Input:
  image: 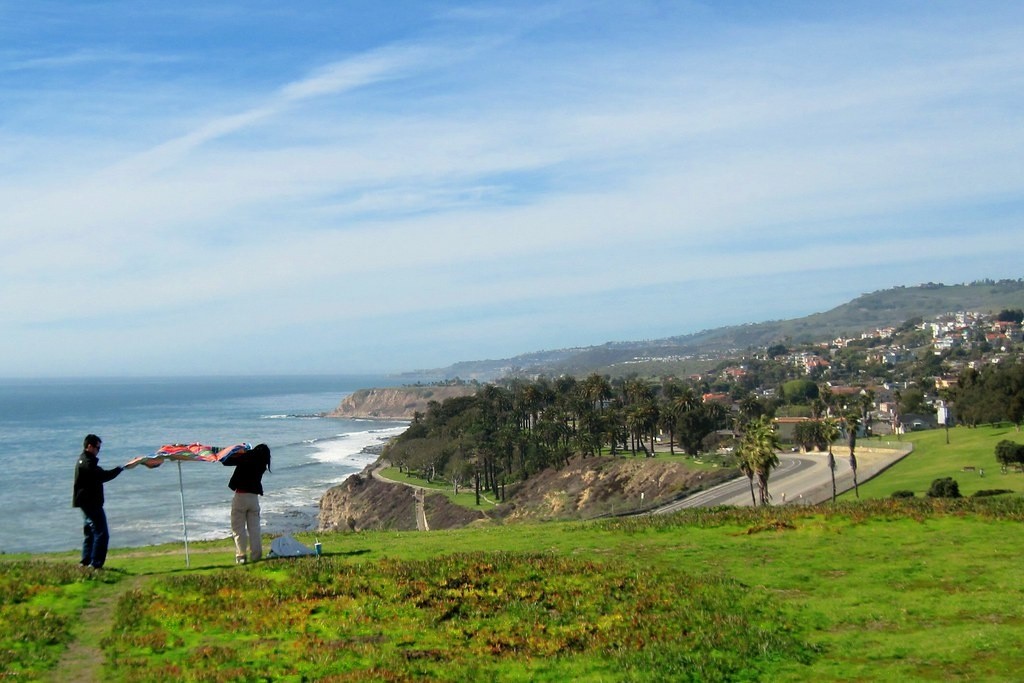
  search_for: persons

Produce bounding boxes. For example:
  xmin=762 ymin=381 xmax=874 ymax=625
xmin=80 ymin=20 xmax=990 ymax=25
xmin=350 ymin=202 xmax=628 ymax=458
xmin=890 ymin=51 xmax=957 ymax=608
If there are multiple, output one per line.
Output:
xmin=220 ymin=444 xmax=272 ymax=564
xmin=72 ymin=434 xmax=126 ymax=571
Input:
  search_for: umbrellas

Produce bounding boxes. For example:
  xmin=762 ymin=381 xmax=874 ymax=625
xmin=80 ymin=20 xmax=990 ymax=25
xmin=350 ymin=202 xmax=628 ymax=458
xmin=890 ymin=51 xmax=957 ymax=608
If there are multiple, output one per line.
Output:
xmin=126 ymin=442 xmax=252 ymax=567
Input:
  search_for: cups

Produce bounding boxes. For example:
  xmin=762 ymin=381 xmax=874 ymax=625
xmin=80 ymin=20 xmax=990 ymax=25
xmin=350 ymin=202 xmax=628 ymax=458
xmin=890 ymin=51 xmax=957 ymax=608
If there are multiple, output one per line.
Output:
xmin=314 ymin=543 xmax=322 ymax=555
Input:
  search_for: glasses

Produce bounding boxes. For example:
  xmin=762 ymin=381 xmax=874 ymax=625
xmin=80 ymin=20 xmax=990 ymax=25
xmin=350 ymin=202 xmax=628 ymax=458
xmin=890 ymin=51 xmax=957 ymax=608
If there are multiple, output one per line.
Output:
xmin=91 ymin=445 xmax=100 ymax=452
